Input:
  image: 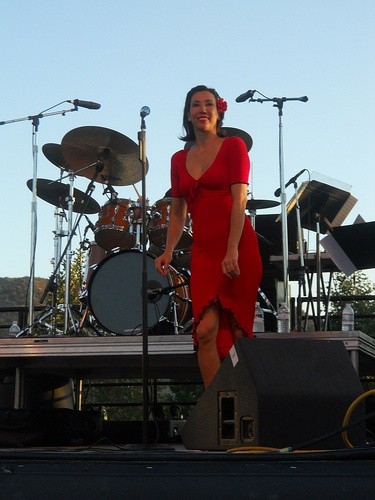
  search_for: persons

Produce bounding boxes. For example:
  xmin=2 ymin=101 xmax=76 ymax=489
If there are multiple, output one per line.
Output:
xmin=155 ymin=85 xmax=261 ymax=393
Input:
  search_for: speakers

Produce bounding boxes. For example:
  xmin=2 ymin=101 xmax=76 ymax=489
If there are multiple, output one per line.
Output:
xmin=178 ymin=336 xmax=367 ymax=452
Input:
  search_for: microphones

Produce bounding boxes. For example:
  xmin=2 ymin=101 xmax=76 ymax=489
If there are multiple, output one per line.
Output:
xmin=85 ymin=163 xmax=104 ymax=194
xmin=235 ymin=90 xmax=256 ymax=103
xmin=147 ymin=289 xmax=161 ymax=300
xmin=140 ymin=106 xmax=150 ymax=117
xmin=101 ymin=175 xmax=118 ymax=196
xmin=274 ymin=169 xmax=305 ymax=197
xmin=85 ymin=214 xmax=99 ymax=234
xmin=66 ymin=99 xmax=101 ymax=110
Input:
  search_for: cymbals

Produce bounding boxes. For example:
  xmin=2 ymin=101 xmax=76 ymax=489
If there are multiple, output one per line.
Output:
xmin=41 ymin=142 xmax=84 ymax=177
xmin=245 ymin=199 xmax=281 ymax=211
xmin=26 ymin=178 xmax=102 ymax=215
xmin=60 ymin=125 xmax=150 ymax=187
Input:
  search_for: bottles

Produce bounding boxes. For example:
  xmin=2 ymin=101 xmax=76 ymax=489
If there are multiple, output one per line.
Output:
xmin=277 ymin=303 xmax=291 ymax=333
xmin=341 ymin=304 xmax=354 ymax=331
xmin=9 ymin=320 xmax=21 ymax=338
xmin=252 ymin=302 xmax=265 ymax=332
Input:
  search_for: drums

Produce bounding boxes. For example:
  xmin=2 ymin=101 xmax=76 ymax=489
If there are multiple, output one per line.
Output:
xmin=160 ymin=249 xmax=191 ymax=268
xmin=94 ymin=197 xmax=143 ymax=252
xmin=87 ymin=247 xmax=190 ymax=336
xmin=85 ymin=240 xmax=116 ymax=290
xmin=146 ymin=198 xmax=194 ymax=250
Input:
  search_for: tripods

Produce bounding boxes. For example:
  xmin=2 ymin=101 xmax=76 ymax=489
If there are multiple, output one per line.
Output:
xmin=15 ymin=158 xmax=102 ymax=339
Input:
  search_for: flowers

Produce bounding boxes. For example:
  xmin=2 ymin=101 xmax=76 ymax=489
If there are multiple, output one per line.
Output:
xmin=216 ymin=98 xmax=227 ymax=111
